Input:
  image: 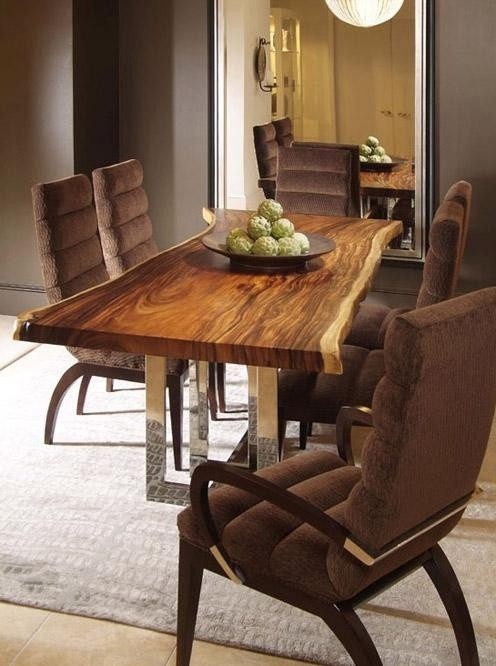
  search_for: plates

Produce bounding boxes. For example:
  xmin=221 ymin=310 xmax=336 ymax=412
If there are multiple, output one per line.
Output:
xmin=359 ymin=157 xmax=409 ymax=172
xmin=202 ymin=232 xmax=336 ymax=269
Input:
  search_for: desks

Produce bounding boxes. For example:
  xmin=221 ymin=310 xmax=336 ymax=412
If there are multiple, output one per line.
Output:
xmin=13 ymin=205 xmax=403 ymax=507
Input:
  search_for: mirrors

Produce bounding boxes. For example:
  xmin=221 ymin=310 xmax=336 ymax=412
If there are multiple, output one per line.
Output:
xmin=205 ymin=1 xmax=440 ymax=270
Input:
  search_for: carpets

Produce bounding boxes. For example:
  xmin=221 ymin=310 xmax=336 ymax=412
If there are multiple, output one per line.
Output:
xmin=0 ymin=342 xmax=496 ymax=666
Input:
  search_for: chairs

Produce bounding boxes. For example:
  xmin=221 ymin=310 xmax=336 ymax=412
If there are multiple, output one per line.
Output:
xmin=251 ymin=117 xmax=414 ymax=246
xmin=91 ymin=159 xmax=227 ymax=412
xmin=31 ymin=174 xmax=217 ymax=472
xmin=177 ymin=287 xmax=496 ymax=666
xmin=277 ymin=180 xmax=471 ymax=463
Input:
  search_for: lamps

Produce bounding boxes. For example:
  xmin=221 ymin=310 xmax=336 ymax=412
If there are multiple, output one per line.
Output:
xmin=325 ymin=0 xmax=404 ymax=28
